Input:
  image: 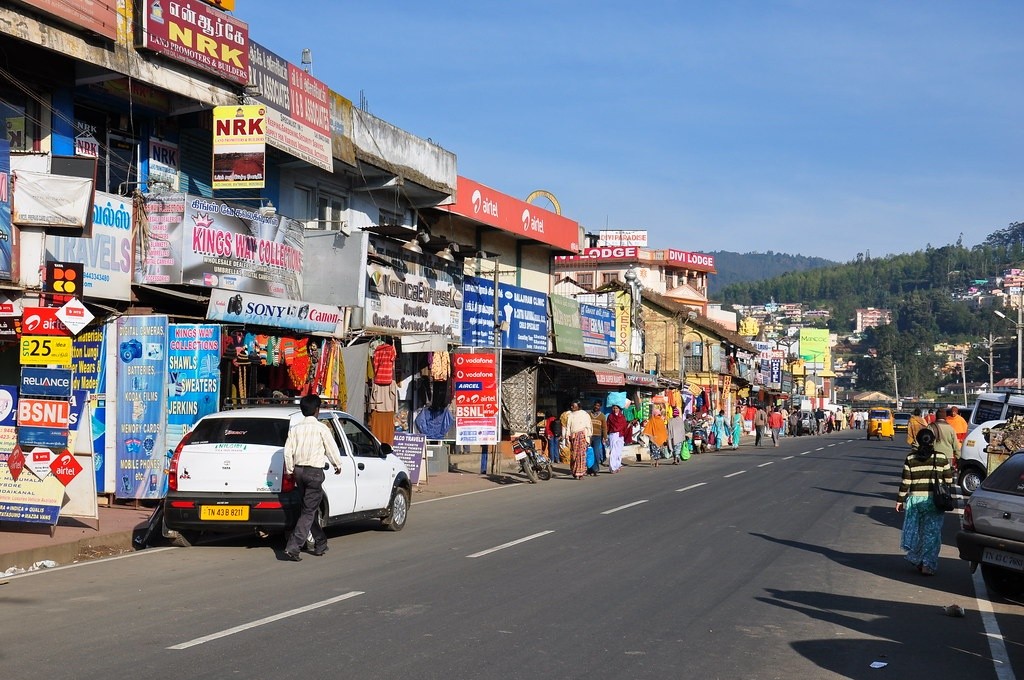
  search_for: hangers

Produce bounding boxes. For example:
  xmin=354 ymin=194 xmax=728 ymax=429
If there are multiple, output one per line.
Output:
xmin=231 ymin=325 xmax=249 ymax=338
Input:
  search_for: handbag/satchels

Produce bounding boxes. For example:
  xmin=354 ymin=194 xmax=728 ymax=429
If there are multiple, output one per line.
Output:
xmin=938 ymin=483 xmax=954 ymax=511
xmin=681 ymin=441 xmax=691 ymax=460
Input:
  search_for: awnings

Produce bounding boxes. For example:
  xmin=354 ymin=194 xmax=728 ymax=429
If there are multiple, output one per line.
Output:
xmin=542 ymin=357 xmax=658 ymax=388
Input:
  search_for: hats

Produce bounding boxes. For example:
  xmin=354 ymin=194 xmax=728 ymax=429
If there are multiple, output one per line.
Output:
xmin=936 ymin=408 xmax=946 ymax=419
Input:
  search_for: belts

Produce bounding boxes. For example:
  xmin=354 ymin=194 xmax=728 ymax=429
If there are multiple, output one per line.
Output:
xmin=295 ymin=464 xmax=323 ymax=470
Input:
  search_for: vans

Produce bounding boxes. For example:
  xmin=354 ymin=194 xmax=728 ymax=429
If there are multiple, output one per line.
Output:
xmin=894 ymin=413 xmax=911 ymax=432
xmin=966 ymin=391 xmax=1023 ymax=436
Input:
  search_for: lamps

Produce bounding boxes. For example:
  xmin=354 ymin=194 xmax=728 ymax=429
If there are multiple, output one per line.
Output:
xmin=462 ymin=251 xmax=487 ymax=271
xmin=295 ymin=219 xmax=352 ymax=237
xmin=402 ymin=232 xmax=430 ymax=255
xmin=214 ymin=198 xmax=276 ymax=216
xmin=435 ymin=243 xmax=459 ymax=263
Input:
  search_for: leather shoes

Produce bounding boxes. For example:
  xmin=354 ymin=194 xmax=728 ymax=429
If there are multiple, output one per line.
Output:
xmin=285 ymin=547 xmax=302 ymax=561
xmin=313 ymin=538 xmax=328 ymax=555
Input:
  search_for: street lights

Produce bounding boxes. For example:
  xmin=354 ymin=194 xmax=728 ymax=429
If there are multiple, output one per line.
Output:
xmin=993 ymin=309 xmax=1023 ymax=390
xmin=979 ymin=357 xmax=993 ymax=393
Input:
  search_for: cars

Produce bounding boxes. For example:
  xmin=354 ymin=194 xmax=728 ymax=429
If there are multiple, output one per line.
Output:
xmin=954 ymin=419 xmax=1024 ymax=575
xmin=797 ymin=410 xmax=817 ymax=435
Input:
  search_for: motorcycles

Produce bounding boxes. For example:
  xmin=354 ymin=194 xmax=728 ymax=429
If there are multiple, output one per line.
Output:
xmin=510 ymin=422 xmax=553 ymax=484
xmin=868 ymin=407 xmax=895 ymax=442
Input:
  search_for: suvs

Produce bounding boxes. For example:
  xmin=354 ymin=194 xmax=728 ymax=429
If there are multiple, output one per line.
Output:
xmin=163 ymin=396 xmax=412 ymax=538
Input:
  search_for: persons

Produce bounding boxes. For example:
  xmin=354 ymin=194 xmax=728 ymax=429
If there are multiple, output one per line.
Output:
xmin=925 ymin=409 xmax=936 ymax=424
xmin=666 ymin=408 xmax=685 ymax=464
xmin=684 ymin=414 xmax=694 ymax=439
xmin=906 ymin=408 xmax=928 ymax=452
xmin=643 ymin=409 xmax=668 ymax=467
xmin=896 ymin=428 xmax=952 ymax=578
xmin=711 ymin=410 xmax=732 ymax=452
xmin=257 ymin=388 xmax=273 ymax=404
xmin=945 ymin=406 xmax=968 ymax=447
xmin=729 ymin=406 xmax=746 ymax=450
xmin=753 ymin=404 xmax=870 ymax=447
xmin=283 ymin=394 xmax=343 ymax=561
xmin=925 ymin=409 xmax=961 ymax=467
xmin=544 ymin=398 xmax=637 ymax=480
